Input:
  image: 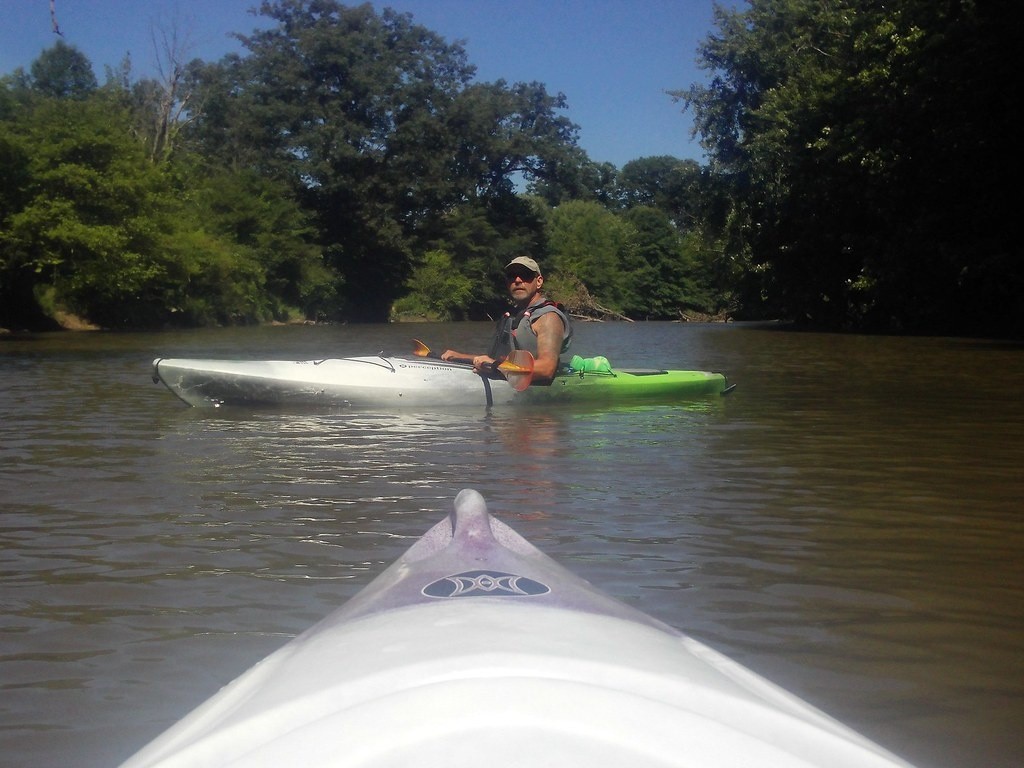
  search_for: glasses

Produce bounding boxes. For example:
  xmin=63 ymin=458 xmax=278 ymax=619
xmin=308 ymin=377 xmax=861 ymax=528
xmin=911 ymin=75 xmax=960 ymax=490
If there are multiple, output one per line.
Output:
xmin=507 ymin=272 xmax=538 ymax=282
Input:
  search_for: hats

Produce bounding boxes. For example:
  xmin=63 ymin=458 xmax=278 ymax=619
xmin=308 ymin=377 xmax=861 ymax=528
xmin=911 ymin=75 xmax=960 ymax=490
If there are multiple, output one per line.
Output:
xmin=504 ymin=256 xmax=541 ymax=276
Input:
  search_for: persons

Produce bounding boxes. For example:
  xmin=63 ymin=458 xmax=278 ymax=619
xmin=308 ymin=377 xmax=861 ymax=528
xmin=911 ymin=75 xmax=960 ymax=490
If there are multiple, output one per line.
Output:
xmin=441 ymin=257 xmax=572 ymax=380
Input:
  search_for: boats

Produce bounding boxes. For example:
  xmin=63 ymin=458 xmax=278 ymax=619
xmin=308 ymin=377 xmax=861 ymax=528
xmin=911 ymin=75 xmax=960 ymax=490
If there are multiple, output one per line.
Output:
xmin=153 ymin=356 xmax=725 ymax=410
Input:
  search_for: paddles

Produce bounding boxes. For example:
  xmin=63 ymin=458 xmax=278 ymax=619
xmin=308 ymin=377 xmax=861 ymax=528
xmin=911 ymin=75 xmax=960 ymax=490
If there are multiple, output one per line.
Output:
xmin=410 ymin=339 xmax=535 ymax=392
